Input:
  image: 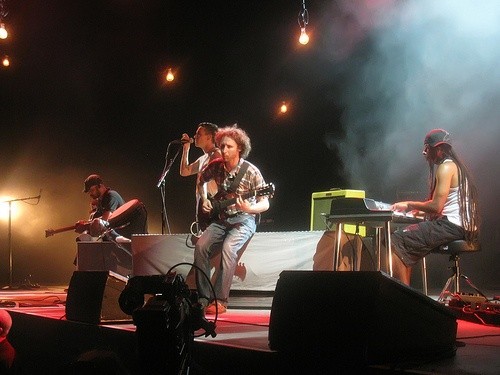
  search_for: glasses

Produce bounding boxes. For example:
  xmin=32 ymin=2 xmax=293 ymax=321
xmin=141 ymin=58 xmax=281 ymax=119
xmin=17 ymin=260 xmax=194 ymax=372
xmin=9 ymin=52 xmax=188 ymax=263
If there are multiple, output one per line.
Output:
xmin=423 ymin=147 xmax=431 ymax=156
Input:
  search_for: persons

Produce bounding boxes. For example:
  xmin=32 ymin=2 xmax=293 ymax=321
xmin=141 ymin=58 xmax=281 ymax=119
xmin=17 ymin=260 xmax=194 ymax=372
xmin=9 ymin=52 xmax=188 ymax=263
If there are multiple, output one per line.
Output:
xmin=0 ymin=309 xmax=20 ymax=375
xmin=193 ymin=127 xmax=270 ymax=317
xmin=390 ymin=127 xmax=481 ymax=293
xmin=181 ymin=122 xmax=224 ymax=235
xmin=64 ymin=175 xmax=124 ymax=293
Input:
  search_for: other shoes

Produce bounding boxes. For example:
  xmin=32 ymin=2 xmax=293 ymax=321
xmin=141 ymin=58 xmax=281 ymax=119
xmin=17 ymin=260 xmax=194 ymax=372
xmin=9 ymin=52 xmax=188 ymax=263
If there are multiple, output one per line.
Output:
xmin=204 ymin=303 xmax=227 ymax=314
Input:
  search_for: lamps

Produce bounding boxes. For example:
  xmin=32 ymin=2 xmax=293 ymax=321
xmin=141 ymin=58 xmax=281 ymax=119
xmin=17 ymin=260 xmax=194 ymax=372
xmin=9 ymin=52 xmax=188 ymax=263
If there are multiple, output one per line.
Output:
xmin=0 ymin=22 xmax=7 ymax=38
xmin=300 ymin=21 xmax=309 ymax=44
xmin=3 ymin=55 xmax=10 ymax=67
xmin=166 ymin=66 xmax=174 ymax=81
xmin=281 ymin=101 xmax=288 ymax=113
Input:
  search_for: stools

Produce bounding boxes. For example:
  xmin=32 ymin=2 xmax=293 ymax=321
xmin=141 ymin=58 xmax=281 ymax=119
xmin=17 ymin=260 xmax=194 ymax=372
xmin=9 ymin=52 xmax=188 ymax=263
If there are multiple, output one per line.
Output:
xmin=434 ymin=241 xmax=488 ymax=307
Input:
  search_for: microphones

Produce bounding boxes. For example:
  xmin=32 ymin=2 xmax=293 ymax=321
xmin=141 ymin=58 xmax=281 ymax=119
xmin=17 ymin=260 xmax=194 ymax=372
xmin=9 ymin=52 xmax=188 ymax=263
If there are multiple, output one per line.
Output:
xmin=172 ymin=137 xmax=194 ymax=145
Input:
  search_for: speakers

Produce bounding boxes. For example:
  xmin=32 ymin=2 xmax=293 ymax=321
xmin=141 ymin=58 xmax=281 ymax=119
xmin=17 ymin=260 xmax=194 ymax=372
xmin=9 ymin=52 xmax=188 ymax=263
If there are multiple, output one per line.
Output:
xmin=267 ymin=271 xmax=459 ymax=367
xmin=66 ymin=270 xmax=134 ymax=324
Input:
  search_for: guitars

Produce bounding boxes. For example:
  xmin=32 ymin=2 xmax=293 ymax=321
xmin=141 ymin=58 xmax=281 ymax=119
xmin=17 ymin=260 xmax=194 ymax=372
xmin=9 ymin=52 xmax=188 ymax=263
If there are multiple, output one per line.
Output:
xmin=45 ymin=198 xmax=147 ymax=238
xmin=198 ymin=182 xmax=275 ymax=229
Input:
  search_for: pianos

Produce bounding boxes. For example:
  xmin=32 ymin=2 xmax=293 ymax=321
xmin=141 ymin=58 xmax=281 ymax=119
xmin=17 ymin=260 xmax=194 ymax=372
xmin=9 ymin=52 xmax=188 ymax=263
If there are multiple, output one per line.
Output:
xmin=330 ymin=197 xmax=428 ymax=223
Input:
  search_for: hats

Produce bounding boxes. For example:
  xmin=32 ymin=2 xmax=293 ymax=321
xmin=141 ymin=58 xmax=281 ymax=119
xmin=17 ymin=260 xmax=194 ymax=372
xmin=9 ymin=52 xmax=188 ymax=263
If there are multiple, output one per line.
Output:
xmin=83 ymin=175 xmax=102 ymax=192
xmin=425 ymin=129 xmax=453 ymax=148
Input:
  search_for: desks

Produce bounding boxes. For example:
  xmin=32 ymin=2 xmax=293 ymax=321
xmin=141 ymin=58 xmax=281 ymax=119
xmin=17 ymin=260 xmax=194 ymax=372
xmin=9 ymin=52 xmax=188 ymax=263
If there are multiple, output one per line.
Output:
xmin=326 ymin=212 xmax=429 ymax=301
xmin=131 ymin=229 xmax=365 ymax=311
